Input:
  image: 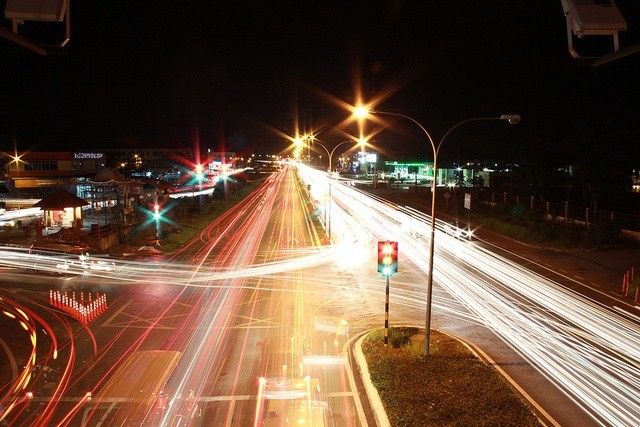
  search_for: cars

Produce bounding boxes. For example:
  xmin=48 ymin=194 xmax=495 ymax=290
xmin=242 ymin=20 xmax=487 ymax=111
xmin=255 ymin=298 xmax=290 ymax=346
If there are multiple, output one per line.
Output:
xmin=444 ymin=220 xmax=475 ymax=240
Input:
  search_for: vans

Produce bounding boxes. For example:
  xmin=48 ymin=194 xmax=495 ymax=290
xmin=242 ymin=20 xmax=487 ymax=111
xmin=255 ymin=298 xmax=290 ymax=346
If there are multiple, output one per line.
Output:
xmin=83 ymin=349 xmax=202 ymax=427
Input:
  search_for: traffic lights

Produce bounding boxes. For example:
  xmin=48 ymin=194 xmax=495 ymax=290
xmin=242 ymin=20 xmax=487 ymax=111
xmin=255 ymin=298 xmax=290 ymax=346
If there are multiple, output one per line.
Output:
xmin=377 ymin=240 xmax=398 ymax=277
xmin=154 ymin=204 xmax=160 ymax=220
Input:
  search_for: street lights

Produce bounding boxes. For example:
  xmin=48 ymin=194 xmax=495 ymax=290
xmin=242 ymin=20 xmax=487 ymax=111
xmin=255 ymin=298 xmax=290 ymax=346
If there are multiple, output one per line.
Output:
xmin=352 ymin=107 xmax=521 ymax=359
xmin=306 ymin=138 xmax=367 ymax=245
